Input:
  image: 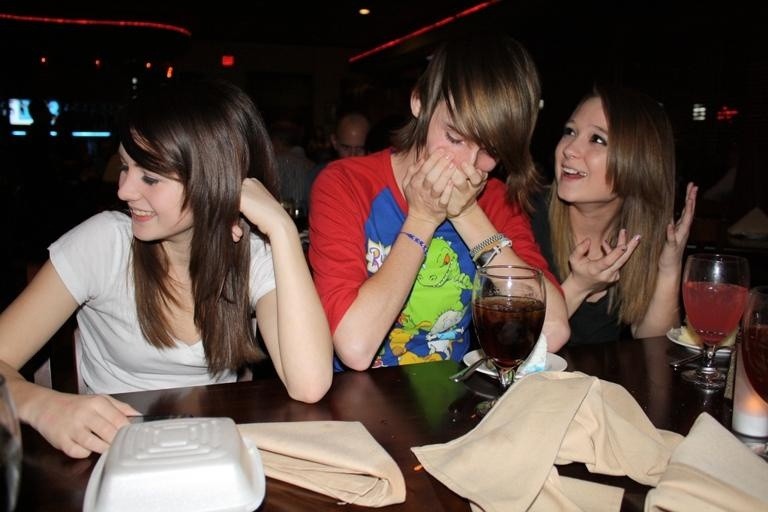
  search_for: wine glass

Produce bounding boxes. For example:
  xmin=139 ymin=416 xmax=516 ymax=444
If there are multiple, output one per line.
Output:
xmin=472 ymin=266 xmax=544 ymax=413
xmin=682 ymin=254 xmax=750 ymax=387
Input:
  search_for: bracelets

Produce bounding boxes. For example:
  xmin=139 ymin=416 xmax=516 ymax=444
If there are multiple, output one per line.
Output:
xmin=469 ymin=233 xmax=512 ymax=268
xmin=399 ymin=231 xmax=429 ymax=256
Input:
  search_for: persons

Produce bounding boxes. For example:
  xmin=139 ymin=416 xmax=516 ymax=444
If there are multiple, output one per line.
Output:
xmin=309 ymin=25 xmax=571 ymax=372
xmin=249 ymin=114 xmax=369 ymax=207
xmin=0 ymin=78 xmax=333 ymax=460
xmin=531 ymin=79 xmax=698 ymax=345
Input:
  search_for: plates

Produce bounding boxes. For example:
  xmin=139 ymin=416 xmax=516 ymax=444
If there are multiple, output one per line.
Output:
xmin=463 ymin=348 xmax=567 ymax=382
xmin=667 ymin=324 xmax=733 ymax=355
